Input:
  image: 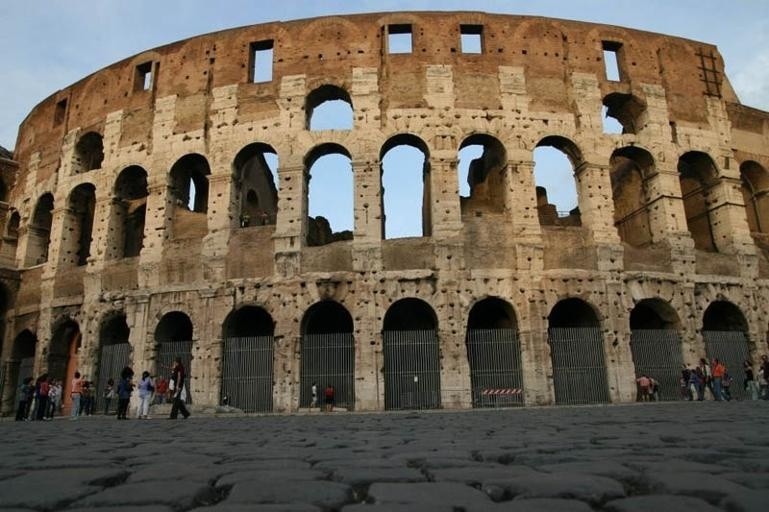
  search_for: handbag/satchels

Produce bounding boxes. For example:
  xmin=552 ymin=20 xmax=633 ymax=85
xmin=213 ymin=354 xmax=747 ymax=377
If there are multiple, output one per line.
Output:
xmin=147 ymin=384 xmax=154 ymax=391
xmin=722 ymin=377 xmax=730 ymax=386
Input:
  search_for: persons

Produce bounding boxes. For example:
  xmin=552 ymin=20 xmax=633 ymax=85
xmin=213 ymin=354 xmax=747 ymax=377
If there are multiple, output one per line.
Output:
xmin=242 ymin=210 xmax=249 ymax=227
xmin=310 ymin=381 xmax=318 ymax=408
xmin=260 ymin=211 xmax=269 ymax=225
xmin=14 ymin=355 xmax=190 ymax=421
xmin=636 ymin=355 xmax=769 ymax=402
xmin=324 ymin=384 xmax=334 ymax=412
